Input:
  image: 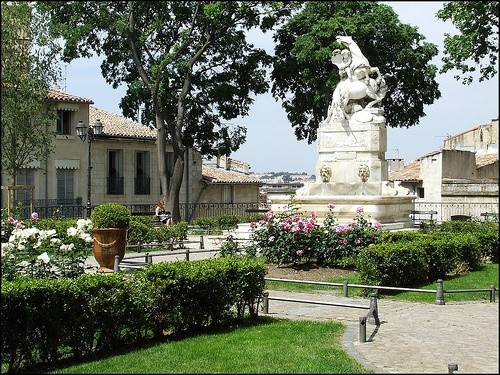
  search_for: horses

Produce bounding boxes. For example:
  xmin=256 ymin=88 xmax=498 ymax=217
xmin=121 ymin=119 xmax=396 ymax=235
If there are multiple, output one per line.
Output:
xmin=323 ymin=35 xmax=386 ymax=124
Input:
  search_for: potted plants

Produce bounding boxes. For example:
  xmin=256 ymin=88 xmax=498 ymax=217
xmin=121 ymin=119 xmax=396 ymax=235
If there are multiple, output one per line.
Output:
xmin=90 ymin=202 xmax=131 ymax=273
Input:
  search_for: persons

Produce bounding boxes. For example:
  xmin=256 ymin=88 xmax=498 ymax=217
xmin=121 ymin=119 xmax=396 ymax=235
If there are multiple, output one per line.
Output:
xmin=153 ymin=199 xmax=174 ymax=227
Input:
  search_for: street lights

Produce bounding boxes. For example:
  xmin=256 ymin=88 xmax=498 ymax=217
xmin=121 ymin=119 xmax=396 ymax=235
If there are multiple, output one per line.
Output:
xmin=75 ymin=118 xmax=105 ymax=230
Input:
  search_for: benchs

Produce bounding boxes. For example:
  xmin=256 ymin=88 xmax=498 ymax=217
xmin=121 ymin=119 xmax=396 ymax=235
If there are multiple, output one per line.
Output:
xmin=410 ymin=210 xmax=437 ymax=226
